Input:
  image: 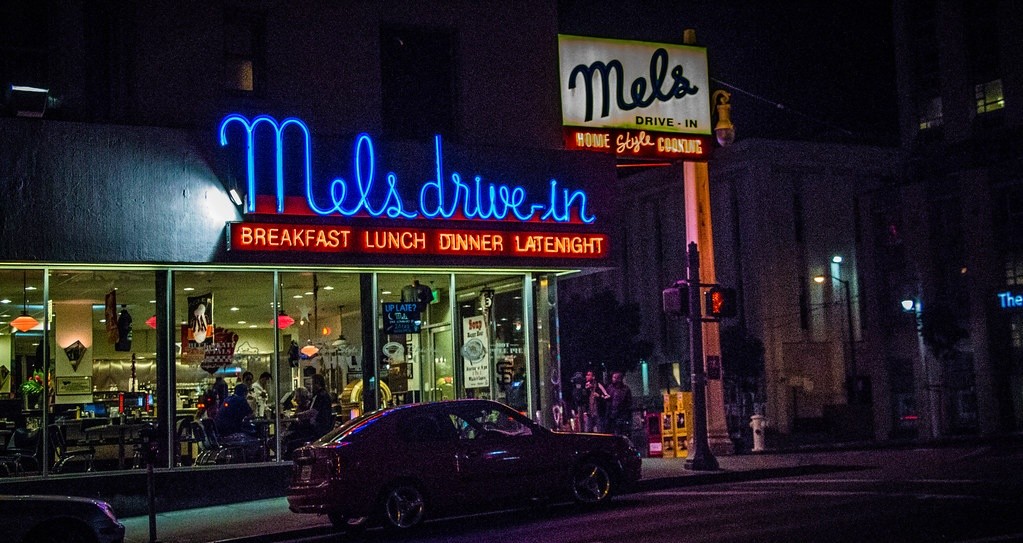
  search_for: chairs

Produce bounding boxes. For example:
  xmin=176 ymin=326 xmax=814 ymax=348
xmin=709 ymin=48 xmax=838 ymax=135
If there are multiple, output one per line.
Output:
xmin=0 ymin=415 xmax=250 ymax=476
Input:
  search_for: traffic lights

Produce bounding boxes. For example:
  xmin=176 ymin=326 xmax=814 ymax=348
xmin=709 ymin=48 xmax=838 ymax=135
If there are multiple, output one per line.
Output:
xmin=706 ymin=287 xmax=737 ymax=319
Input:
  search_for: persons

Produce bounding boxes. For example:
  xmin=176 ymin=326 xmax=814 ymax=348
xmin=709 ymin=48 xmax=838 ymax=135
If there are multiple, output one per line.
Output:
xmin=287 ymin=339 xmax=299 ymax=367
xmin=603 ymin=372 xmax=633 ymax=435
xmin=506 ymin=374 xmax=527 ymax=412
xmin=267 ymin=387 xmax=309 ymax=454
xmin=195 ymin=387 xmax=220 ymax=449
xmin=283 ymin=374 xmax=335 ymax=453
xmin=578 ymin=369 xmax=612 ymax=432
xmin=214 ymin=383 xmax=260 ymax=450
xmin=246 ymin=372 xmax=273 ymax=417
xmin=242 ymin=371 xmax=253 ymax=393
xmin=400 ymin=280 xmax=434 ymax=312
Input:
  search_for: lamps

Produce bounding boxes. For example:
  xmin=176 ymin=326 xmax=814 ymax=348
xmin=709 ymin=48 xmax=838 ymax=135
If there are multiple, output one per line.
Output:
xmin=145 ymin=315 xmax=156 ymax=330
xmin=300 ymin=314 xmax=320 ymax=358
xmin=9 ymin=270 xmax=41 ymax=333
xmin=332 ymin=305 xmax=351 ymax=349
xmin=270 ymin=273 xmax=295 ymax=332
xmin=64 ymin=340 xmax=87 ymax=373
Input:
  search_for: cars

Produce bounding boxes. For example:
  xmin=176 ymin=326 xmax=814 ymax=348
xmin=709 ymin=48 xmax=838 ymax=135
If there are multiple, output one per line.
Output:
xmin=0 ymin=493 xmax=126 ymax=543
xmin=288 ymin=397 xmax=643 ymax=537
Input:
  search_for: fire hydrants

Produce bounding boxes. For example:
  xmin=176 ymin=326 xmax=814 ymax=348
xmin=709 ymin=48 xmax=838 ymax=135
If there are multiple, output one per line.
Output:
xmin=750 ymin=412 xmax=770 ymax=454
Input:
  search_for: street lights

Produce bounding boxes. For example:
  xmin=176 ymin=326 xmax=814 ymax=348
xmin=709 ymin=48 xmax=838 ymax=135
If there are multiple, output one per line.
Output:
xmin=813 ymin=273 xmax=858 ymax=381
xmin=683 ymin=87 xmax=735 ymax=455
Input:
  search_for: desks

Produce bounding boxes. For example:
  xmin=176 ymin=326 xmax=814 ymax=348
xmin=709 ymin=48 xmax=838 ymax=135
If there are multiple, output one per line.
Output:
xmin=251 ymin=418 xmax=296 ymax=460
xmin=86 ymin=424 xmax=145 ymax=465
